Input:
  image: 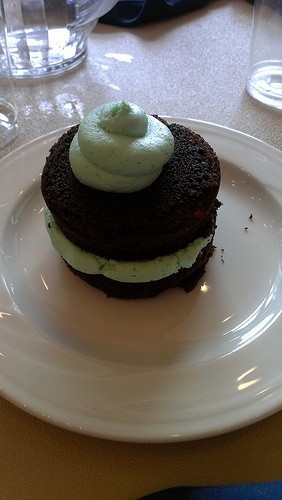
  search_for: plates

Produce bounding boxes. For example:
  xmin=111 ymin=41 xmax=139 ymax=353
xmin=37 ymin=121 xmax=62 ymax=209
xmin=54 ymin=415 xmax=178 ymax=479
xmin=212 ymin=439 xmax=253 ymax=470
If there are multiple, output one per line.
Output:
xmin=0 ymin=115 xmax=282 ymax=445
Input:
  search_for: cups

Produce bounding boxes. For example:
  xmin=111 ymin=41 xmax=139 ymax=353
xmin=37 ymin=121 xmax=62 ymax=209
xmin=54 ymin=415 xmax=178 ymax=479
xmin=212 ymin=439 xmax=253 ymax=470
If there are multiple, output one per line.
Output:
xmin=0 ymin=13 xmax=20 ymax=148
xmin=0 ymin=0 xmax=117 ymax=78
xmin=244 ymin=0 xmax=282 ymax=109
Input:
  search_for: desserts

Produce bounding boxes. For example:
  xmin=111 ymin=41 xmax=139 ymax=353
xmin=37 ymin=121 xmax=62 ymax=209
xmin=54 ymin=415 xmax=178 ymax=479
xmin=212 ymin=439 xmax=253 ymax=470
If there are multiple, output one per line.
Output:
xmin=41 ymin=100 xmax=224 ymax=300
